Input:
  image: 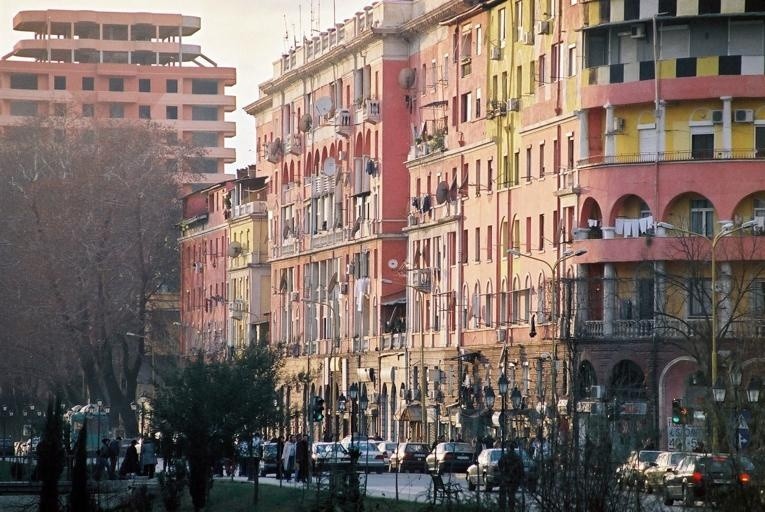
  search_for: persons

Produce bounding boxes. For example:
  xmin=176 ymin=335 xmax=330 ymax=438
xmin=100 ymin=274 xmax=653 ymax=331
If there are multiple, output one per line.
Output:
xmin=368 ymin=432 xmax=384 ymax=441
xmin=95 ymin=433 xmax=310 ymax=485
xmin=431 ymin=426 xmax=549 ymax=512
xmin=323 ymin=426 xmax=333 ymax=442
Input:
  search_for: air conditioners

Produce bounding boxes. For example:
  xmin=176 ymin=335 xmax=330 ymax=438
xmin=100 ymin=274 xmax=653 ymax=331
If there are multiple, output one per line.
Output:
xmin=407 ymin=215 xmax=417 ymax=227
xmin=536 ymin=20 xmax=549 ymax=34
xmin=711 ymin=109 xmax=733 ymax=123
xmin=490 ymin=47 xmax=500 ymax=60
xmin=507 ymin=99 xmax=519 ymax=111
xmin=630 ymin=23 xmax=646 ymax=39
xmin=522 ymin=32 xmax=534 ymax=45
xmin=734 ymin=109 xmax=753 ymax=123
xmin=590 ymin=385 xmax=605 ymax=399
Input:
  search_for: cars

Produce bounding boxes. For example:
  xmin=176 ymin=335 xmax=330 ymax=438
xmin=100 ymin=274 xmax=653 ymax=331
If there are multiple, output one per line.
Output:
xmin=612 ymin=446 xmax=758 ymax=510
xmin=0 ymin=436 xmax=41 ymax=457
xmin=388 ymin=443 xmax=433 ymax=475
xmin=376 ymin=441 xmax=398 ymax=470
xmin=424 ymin=442 xmax=476 ymax=476
xmin=465 ymin=447 xmax=540 ymax=495
xmin=115 ymin=426 xmax=298 ymax=480
xmin=338 ymin=441 xmax=385 ymax=475
xmin=310 ymin=441 xmax=353 ymax=479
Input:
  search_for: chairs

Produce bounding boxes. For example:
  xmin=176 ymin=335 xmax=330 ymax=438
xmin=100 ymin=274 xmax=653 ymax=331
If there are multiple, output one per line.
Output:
xmin=430 ymin=473 xmax=462 ymax=506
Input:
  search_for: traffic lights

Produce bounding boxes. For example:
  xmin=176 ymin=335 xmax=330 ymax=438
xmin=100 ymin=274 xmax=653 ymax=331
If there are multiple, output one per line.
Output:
xmin=672 ymin=399 xmax=682 ymax=425
xmin=682 ymin=408 xmax=693 ymax=424
xmin=314 ymin=397 xmax=325 ymax=422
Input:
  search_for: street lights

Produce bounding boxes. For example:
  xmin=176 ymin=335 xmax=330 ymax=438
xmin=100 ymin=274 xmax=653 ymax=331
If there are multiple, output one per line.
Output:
xmin=299 ymin=297 xmax=340 ymax=440
xmin=336 ymin=382 xmax=370 ymax=435
xmin=0 ymin=400 xmax=14 ymax=461
xmin=130 ymin=392 xmax=155 ymax=474
xmin=377 ymin=276 xmax=429 ymax=450
xmin=94 ymin=400 xmax=104 ymax=511
xmin=712 ymin=356 xmax=761 ymax=512
xmin=173 ymin=320 xmax=203 ymax=353
xmin=505 ymin=247 xmax=588 ymax=458
xmin=125 ymin=331 xmax=157 ymax=393
xmin=483 ymin=371 xmax=524 ymax=511
xmin=238 ymin=308 xmax=264 ymax=348
xmin=23 ymin=404 xmax=42 ymax=483
xmin=654 ymin=215 xmax=762 ymax=512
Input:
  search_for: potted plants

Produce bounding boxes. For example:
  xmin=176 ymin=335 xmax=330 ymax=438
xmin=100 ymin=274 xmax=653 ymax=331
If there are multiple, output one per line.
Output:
xmin=415 ymin=130 xmax=445 ymax=154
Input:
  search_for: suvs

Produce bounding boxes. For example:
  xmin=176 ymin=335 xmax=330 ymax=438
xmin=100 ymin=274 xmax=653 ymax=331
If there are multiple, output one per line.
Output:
xmin=340 ymin=433 xmax=383 ymax=442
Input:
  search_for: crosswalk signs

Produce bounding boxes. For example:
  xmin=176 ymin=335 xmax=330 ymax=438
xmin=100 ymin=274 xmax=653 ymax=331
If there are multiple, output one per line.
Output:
xmin=729 ymin=411 xmax=754 ymax=434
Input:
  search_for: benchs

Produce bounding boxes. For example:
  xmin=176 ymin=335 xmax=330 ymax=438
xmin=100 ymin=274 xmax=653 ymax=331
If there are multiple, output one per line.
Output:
xmin=128 ymin=480 xmax=177 ymax=497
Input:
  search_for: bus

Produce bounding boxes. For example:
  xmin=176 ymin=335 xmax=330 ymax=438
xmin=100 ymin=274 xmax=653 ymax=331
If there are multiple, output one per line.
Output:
xmin=60 ymin=405 xmax=116 ymax=453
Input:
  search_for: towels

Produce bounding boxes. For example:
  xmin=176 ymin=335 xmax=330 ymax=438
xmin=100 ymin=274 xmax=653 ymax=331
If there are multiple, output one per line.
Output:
xmin=587 ymin=218 xmax=597 ymax=227
xmin=614 ymin=216 xmax=653 ymax=238
